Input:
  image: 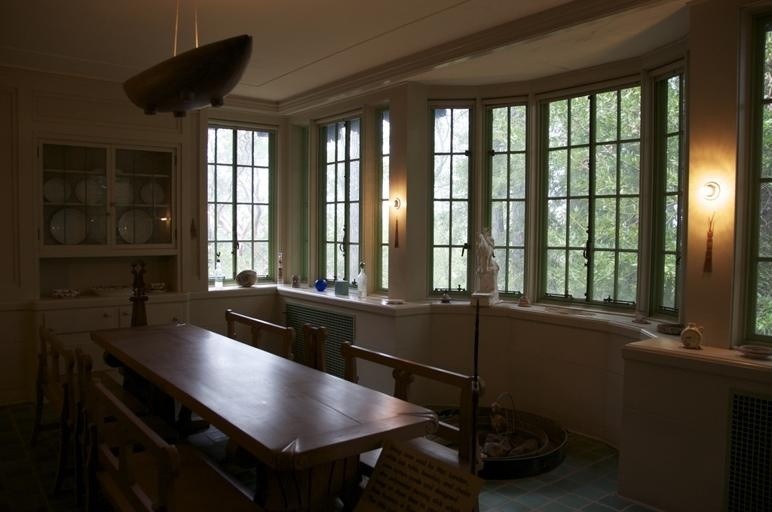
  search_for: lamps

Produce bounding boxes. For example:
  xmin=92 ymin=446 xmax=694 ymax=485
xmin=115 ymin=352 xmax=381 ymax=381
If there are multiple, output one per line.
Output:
xmin=123 ymin=0 xmax=253 ymax=119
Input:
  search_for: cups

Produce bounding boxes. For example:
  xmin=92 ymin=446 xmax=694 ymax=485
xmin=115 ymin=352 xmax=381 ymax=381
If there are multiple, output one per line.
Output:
xmin=335 ymin=280 xmax=349 ymax=296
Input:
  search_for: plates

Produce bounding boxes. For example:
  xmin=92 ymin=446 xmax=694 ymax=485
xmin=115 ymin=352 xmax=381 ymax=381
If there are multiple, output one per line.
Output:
xmin=118 ymin=208 xmax=154 ymax=244
xmin=49 ymin=207 xmax=89 ymax=244
xmin=43 ymin=176 xmax=165 ymax=204
xmin=738 ymin=344 xmax=772 ymax=358
xmin=146 ymin=288 xmax=169 ymax=293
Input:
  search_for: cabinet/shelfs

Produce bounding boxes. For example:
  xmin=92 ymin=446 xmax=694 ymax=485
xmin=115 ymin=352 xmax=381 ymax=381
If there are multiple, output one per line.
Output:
xmin=35 ymin=293 xmax=190 ymax=429
xmin=35 ymin=134 xmax=182 ymax=256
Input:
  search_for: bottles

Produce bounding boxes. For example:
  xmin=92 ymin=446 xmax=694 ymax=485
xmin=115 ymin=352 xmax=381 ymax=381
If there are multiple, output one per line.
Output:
xmin=357 ymin=262 xmax=367 ymax=300
xmin=291 ymin=272 xmax=301 ymax=288
xmin=214 ymin=259 xmax=225 ymax=288
